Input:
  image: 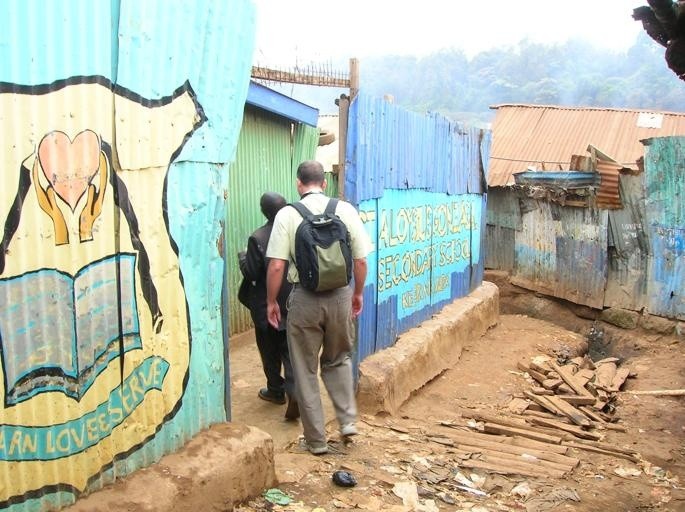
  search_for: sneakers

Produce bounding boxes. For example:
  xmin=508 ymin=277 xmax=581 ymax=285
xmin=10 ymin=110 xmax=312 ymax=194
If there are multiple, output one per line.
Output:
xmin=340 ymin=423 xmax=358 ymax=437
xmin=299 ymin=435 xmax=329 ymax=455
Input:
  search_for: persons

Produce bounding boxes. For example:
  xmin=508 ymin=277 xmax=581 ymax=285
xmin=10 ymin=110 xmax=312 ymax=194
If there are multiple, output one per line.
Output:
xmin=266 ymin=160 xmax=368 ymax=455
xmin=33 ymin=151 xmax=107 ymax=246
xmin=237 ymin=192 xmax=300 ymax=422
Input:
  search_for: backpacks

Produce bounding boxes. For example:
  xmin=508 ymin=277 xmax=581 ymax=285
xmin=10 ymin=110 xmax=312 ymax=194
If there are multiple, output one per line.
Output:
xmin=286 ymin=197 xmax=352 ymax=292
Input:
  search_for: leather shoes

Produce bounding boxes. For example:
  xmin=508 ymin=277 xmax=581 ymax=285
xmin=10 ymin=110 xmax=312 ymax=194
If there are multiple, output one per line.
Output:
xmin=258 ymin=388 xmax=286 ymax=405
xmin=286 ymin=393 xmax=300 ymax=419
